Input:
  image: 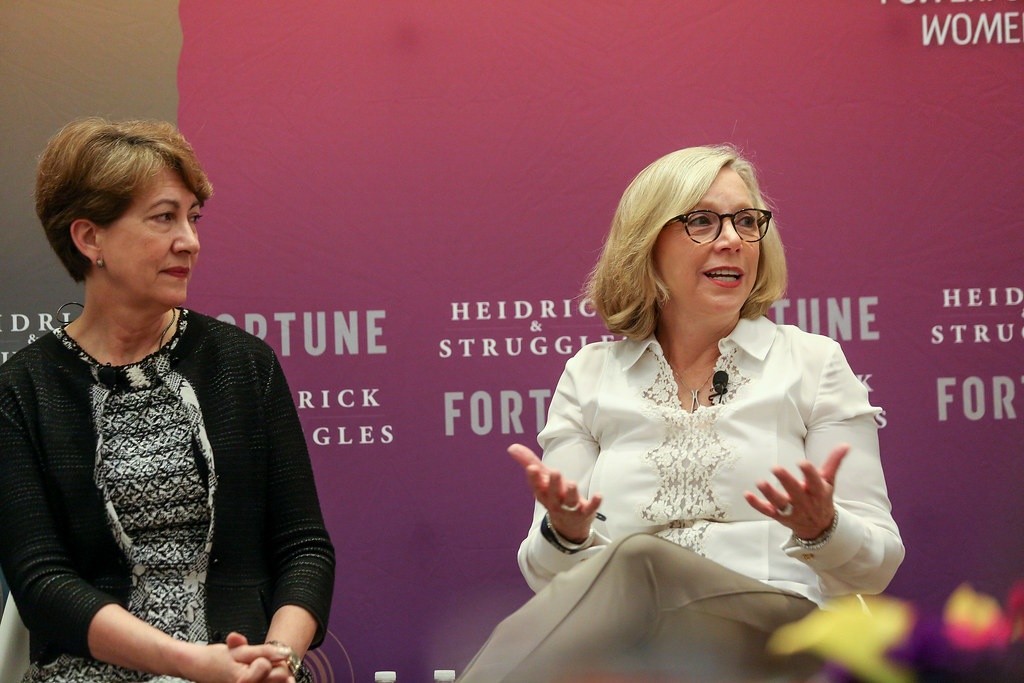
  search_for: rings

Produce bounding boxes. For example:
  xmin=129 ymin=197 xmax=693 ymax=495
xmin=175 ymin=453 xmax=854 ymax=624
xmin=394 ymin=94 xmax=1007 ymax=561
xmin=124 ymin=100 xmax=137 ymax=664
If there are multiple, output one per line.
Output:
xmin=561 ymin=501 xmax=581 ymax=512
xmin=776 ymin=502 xmax=792 ymax=516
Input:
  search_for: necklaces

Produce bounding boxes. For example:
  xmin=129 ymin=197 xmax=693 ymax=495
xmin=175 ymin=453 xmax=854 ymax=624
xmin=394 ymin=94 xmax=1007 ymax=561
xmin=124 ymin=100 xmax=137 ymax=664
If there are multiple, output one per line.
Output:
xmin=667 ymin=360 xmax=720 ymax=414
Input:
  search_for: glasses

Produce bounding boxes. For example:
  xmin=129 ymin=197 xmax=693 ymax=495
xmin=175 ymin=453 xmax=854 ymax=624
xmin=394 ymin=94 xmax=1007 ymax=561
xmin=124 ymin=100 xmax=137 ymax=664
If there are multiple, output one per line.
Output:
xmin=664 ymin=208 xmax=772 ymax=244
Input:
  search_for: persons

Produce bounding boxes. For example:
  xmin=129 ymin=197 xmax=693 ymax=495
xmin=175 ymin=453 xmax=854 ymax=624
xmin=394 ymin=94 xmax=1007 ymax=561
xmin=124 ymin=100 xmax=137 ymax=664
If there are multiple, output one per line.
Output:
xmin=454 ymin=145 xmax=906 ymax=683
xmin=0 ymin=118 xmax=335 ymax=683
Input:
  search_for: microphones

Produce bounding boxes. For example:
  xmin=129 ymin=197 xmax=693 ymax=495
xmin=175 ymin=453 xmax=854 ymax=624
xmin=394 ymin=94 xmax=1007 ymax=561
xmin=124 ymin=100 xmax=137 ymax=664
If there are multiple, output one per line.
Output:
xmin=98 ymin=366 xmax=116 ymax=387
xmin=713 ymin=371 xmax=728 ymax=394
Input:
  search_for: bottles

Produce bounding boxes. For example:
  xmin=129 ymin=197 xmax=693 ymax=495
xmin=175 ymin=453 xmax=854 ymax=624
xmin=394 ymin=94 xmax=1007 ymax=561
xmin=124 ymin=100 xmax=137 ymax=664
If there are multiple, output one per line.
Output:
xmin=433 ymin=669 xmax=456 ymax=683
xmin=374 ymin=671 xmax=397 ymax=683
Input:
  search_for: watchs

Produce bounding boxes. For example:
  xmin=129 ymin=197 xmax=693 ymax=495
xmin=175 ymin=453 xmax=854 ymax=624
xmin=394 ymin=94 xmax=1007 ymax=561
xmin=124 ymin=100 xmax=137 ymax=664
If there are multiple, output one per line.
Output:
xmin=265 ymin=641 xmax=302 ymax=677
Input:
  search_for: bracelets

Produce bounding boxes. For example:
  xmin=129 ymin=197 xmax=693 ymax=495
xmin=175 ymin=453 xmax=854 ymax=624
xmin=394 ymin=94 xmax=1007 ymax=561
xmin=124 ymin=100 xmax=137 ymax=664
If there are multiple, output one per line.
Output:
xmin=792 ymin=510 xmax=839 ymax=550
